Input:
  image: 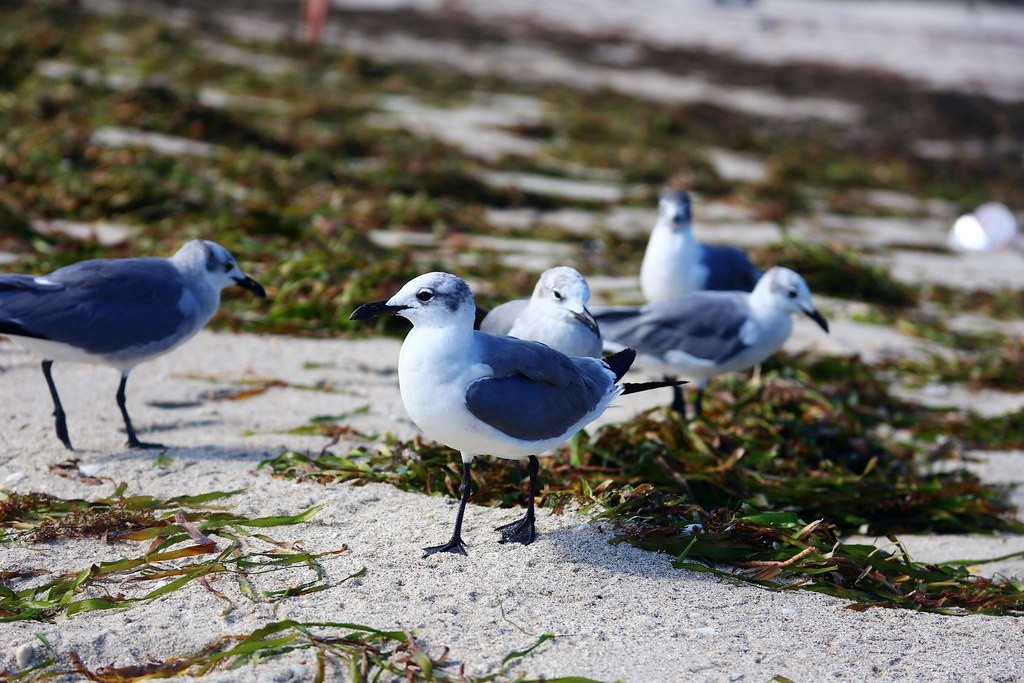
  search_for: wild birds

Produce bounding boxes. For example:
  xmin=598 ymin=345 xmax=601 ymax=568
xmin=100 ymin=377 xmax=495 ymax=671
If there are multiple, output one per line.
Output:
xmin=347 ymin=189 xmax=832 ymax=561
xmin=1 ymin=239 xmax=267 ymax=455
